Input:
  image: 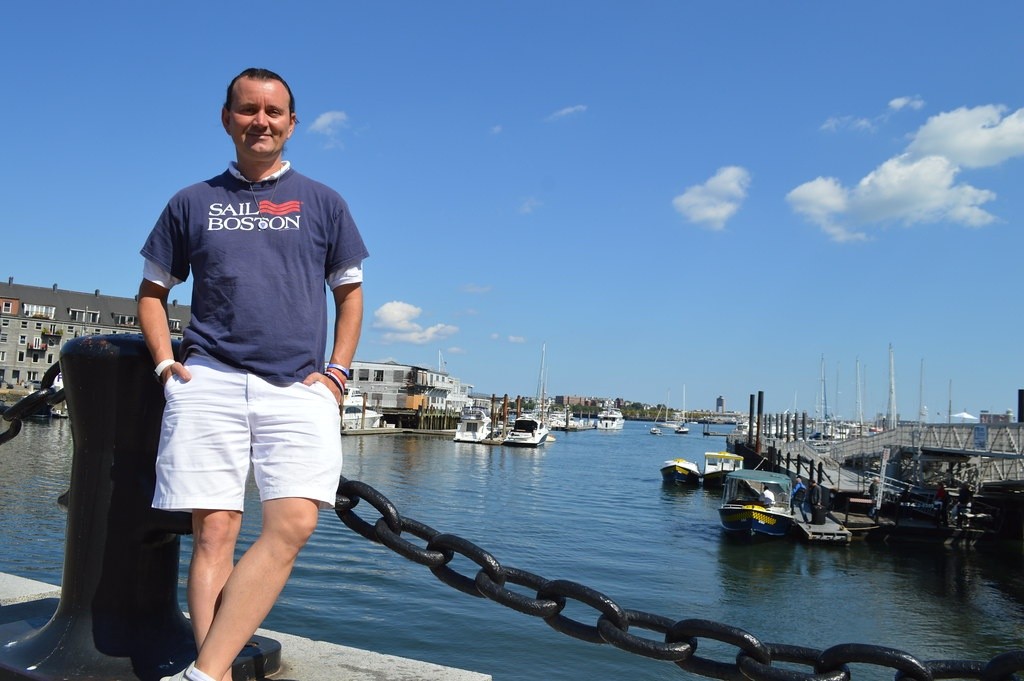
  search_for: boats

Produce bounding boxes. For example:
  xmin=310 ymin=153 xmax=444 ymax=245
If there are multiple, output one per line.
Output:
xmin=510 ymin=399 xmax=625 ymax=430
xmin=453 ymin=406 xmax=492 ymax=442
xmin=650 ymin=427 xmax=662 ymax=435
xmin=698 ymin=416 xmax=737 ymax=425
xmin=503 ymin=414 xmax=548 ymax=448
xmin=718 ymin=468 xmax=794 ymax=535
xmin=21 ymin=372 xmax=69 ymax=424
xmin=660 ymin=458 xmax=702 ymax=484
xmin=341 ymin=383 xmax=384 ymax=430
xmin=703 ymin=451 xmax=744 ymax=483
xmin=734 ymin=341 xmax=927 ymax=442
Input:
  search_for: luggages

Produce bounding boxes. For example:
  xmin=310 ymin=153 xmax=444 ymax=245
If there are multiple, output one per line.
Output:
xmin=811 ymin=506 xmax=826 ymax=524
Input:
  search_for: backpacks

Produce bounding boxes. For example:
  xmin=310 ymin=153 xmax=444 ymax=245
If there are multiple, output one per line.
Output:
xmin=794 ymin=483 xmax=806 ymax=502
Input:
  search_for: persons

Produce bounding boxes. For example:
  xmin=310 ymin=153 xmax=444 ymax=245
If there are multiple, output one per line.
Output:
xmin=138 ymin=69 xmax=370 ymax=681
xmin=868 ymin=477 xmax=879 ymax=517
xmin=809 ymin=480 xmax=821 ymax=523
xmin=958 ymin=482 xmax=975 ymax=527
xmin=932 ymin=483 xmax=951 ymax=526
xmin=790 ymin=477 xmax=808 ymax=522
xmin=760 ymin=486 xmax=776 ymax=507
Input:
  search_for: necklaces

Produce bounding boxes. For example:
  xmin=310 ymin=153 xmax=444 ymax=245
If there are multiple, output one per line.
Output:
xmin=243 ymin=166 xmax=282 ymax=229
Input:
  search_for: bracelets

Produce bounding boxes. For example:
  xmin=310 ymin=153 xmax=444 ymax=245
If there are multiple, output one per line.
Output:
xmin=324 ymin=364 xmax=350 ymax=395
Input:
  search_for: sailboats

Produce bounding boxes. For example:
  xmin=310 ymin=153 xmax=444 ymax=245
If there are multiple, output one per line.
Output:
xmin=532 ymin=343 xmax=556 ymax=442
xmin=675 ymin=383 xmax=689 ymax=434
xmin=654 ymin=390 xmax=680 ymax=428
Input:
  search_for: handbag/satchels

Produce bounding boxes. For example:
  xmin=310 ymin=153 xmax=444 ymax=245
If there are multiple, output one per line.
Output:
xmin=934 ymin=501 xmax=943 ymax=510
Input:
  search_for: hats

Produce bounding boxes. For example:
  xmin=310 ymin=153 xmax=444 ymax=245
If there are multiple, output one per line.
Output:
xmin=872 ymin=477 xmax=879 ymax=482
xmin=796 ymin=474 xmax=802 ymax=479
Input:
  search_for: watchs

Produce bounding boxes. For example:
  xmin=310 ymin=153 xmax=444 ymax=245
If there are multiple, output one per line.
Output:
xmin=153 ymin=359 xmax=175 ymax=385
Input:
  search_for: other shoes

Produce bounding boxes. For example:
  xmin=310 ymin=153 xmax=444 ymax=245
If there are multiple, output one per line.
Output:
xmin=160 ymin=660 xmax=204 ymax=681
